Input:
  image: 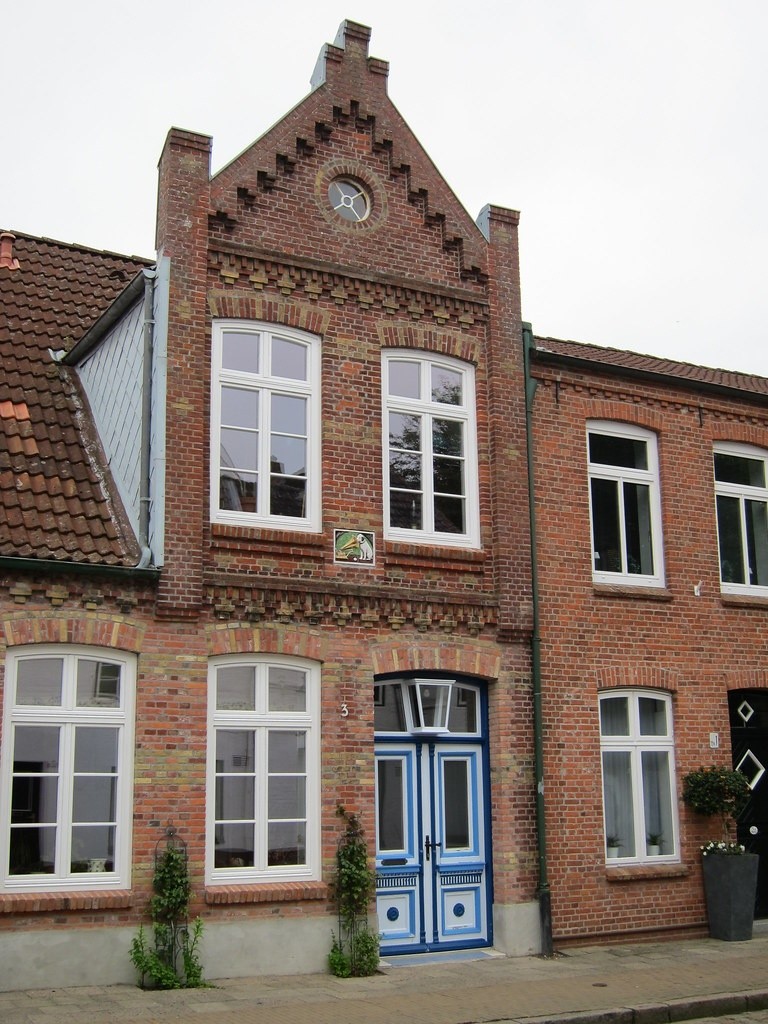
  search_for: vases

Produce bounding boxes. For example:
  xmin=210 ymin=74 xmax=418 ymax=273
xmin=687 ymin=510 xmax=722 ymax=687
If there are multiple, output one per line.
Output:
xmin=701 ymin=851 xmax=759 ymax=943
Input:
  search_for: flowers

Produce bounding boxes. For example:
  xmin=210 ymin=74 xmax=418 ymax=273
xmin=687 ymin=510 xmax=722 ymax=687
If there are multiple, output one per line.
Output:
xmin=683 ymin=764 xmax=751 ymax=857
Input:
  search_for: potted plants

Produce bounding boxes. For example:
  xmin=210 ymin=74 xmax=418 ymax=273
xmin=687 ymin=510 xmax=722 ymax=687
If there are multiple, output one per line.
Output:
xmin=605 ymin=834 xmax=625 ymax=858
xmin=646 ymin=831 xmax=665 ymax=857
